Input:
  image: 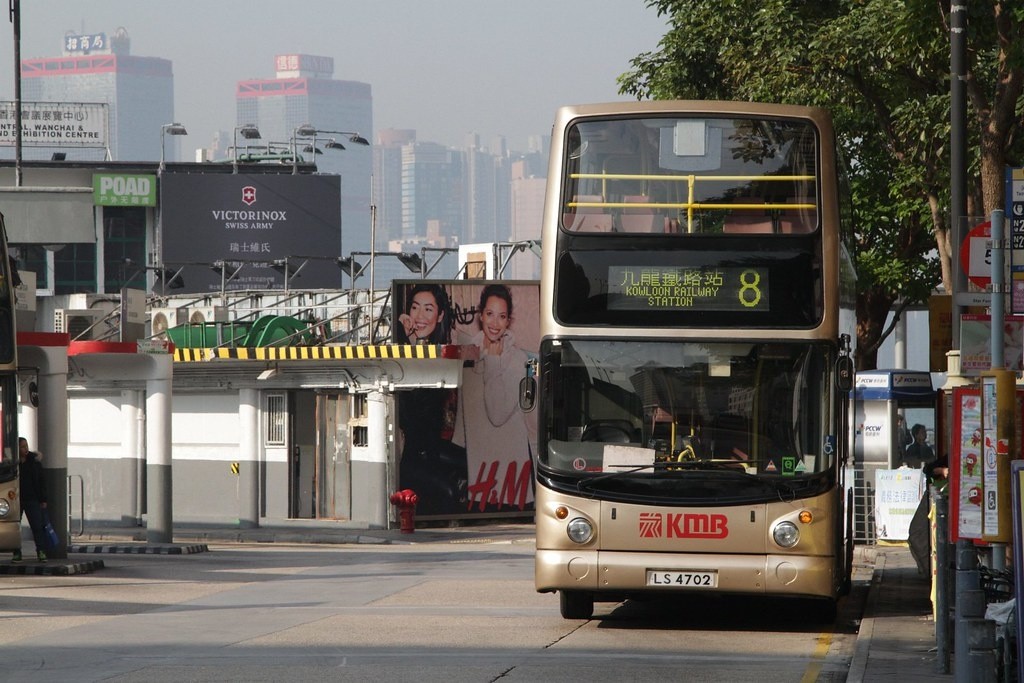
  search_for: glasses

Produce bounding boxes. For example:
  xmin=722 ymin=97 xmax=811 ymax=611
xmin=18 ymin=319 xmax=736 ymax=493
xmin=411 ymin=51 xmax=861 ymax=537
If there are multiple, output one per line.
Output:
xmin=19 ymin=443 xmax=28 ymax=449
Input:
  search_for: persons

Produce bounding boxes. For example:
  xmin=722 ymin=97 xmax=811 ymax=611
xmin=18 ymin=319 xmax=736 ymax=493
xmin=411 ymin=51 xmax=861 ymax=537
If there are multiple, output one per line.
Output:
xmin=12 ymin=436 xmax=58 ymax=563
xmin=905 ymin=425 xmax=933 ymax=473
xmin=897 ymin=415 xmax=912 ymax=454
xmin=396 ymin=284 xmax=534 ymax=512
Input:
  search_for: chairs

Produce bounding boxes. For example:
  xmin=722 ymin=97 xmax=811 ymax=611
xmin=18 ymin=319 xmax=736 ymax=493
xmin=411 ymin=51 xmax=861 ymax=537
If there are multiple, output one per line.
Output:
xmin=778 ymin=215 xmax=817 ymax=233
xmin=616 ymin=196 xmax=677 ymax=233
xmin=562 ymin=194 xmax=615 ymax=233
xmin=732 ymin=197 xmax=767 ymax=217
xmin=786 ymin=197 xmax=816 ymax=215
xmin=723 ymin=216 xmax=774 ymax=233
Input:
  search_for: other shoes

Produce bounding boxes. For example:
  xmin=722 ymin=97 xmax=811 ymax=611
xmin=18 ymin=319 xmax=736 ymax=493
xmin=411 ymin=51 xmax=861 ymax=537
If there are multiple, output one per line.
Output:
xmin=11 ymin=552 xmax=22 ymax=563
xmin=38 ymin=551 xmax=48 ymax=563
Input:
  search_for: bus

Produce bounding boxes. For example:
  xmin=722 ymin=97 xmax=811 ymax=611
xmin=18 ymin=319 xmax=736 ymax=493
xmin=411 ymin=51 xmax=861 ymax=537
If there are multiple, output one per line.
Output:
xmin=535 ymin=102 xmax=854 ymax=619
xmin=0 ymin=212 xmax=39 ymax=557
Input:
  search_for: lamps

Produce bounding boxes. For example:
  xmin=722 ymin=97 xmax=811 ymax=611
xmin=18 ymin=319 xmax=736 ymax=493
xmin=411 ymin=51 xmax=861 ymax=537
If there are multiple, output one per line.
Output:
xmin=285 ymin=256 xmax=363 ymax=289
xmin=209 ymin=259 xmax=249 ymax=291
xmin=265 ymin=257 xmax=302 ymax=291
xmin=351 ymin=251 xmax=427 ymax=289
xmin=122 ymin=265 xmax=186 ymax=291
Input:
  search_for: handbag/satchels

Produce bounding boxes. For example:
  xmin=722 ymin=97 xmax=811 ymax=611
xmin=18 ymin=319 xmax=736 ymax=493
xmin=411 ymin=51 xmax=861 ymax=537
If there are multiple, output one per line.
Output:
xmin=43 ymin=522 xmax=59 ymax=549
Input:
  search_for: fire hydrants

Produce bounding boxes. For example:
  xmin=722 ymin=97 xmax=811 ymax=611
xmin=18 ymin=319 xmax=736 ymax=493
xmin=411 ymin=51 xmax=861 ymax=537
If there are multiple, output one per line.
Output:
xmin=389 ymin=488 xmax=418 ymax=535
